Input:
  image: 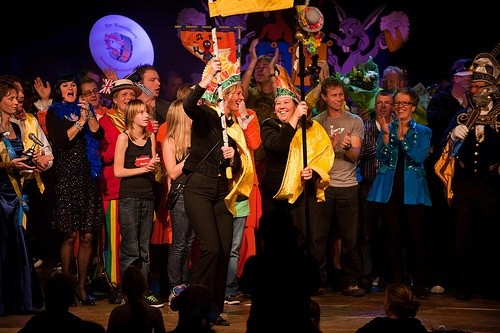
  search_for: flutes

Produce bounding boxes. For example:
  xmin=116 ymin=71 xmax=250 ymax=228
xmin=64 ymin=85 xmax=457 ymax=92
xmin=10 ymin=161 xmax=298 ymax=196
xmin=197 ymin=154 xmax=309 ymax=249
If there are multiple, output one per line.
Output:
xmin=439 ymin=108 xmax=479 ymax=174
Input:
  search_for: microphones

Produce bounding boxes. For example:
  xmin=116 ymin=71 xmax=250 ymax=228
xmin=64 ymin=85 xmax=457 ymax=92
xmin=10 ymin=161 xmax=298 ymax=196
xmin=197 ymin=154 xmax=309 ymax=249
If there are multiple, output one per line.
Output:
xmin=28 ymin=133 xmax=44 ymax=147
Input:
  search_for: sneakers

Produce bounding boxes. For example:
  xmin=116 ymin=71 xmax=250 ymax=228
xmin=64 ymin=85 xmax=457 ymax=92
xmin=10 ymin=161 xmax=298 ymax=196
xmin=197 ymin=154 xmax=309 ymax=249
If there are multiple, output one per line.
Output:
xmin=143 ymin=294 xmax=165 ymax=308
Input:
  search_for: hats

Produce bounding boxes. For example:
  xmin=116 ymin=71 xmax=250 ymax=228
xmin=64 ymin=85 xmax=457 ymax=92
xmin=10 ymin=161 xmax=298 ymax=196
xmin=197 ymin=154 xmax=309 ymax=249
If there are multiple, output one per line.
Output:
xmin=299 ymin=7 xmax=324 ymax=32
xmin=99 ymin=78 xmax=142 ymax=101
xmin=274 ymin=64 xmax=300 ymax=107
xmin=201 ymin=50 xmax=241 ymax=104
xmin=470 ymin=53 xmax=500 ymax=88
xmin=257 ymin=55 xmax=280 ymax=77
xmin=450 ymin=59 xmax=474 ymax=76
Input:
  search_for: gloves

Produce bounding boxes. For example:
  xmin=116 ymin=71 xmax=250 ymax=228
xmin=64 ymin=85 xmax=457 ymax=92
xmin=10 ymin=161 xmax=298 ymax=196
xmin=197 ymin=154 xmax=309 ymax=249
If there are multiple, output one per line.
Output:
xmin=451 ymin=125 xmax=469 ymax=141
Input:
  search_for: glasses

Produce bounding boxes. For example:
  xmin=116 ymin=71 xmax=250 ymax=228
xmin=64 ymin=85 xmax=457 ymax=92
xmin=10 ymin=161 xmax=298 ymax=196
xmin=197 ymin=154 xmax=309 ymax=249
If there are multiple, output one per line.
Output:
xmin=375 ymin=100 xmax=394 ymax=106
xmin=470 ymin=84 xmax=491 ymax=89
xmin=393 ymin=101 xmax=414 ymax=105
xmin=82 ymin=88 xmax=100 ymax=97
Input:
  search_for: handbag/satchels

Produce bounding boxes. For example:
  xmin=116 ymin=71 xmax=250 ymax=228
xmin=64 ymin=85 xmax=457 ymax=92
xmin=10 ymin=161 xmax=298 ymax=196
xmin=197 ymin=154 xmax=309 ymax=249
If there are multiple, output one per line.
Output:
xmin=165 ymin=183 xmax=187 ymax=210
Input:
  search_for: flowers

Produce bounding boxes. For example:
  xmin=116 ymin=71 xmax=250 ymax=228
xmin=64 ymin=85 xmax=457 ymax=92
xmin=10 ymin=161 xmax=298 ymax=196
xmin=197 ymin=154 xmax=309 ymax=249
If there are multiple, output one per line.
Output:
xmin=337 ymin=60 xmax=378 ymax=93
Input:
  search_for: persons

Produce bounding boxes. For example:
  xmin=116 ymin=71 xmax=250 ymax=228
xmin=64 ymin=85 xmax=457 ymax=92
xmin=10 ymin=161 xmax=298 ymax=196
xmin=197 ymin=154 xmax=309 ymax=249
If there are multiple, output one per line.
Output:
xmin=18 ymin=260 xmax=427 ymax=333
xmin=0 ymin=37 xmax=500 ymax=299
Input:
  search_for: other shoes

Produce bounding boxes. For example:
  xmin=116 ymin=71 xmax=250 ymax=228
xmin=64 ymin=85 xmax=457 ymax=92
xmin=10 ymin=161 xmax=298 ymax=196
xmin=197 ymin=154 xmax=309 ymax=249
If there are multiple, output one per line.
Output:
xmin=71 ymin=296 xmax=97 ymax=307
xmin=22 ymin=308 xmax=43 ymax=315
xmin=211 ymin=315 xmax=231 ymax=326
xmin=345 ymin=284 xmax=365 ymax=297
xmin=224 ymin=295 xmax=240 ymax=304
xmin=110 ymin=293 xmax=125 ymax=304
